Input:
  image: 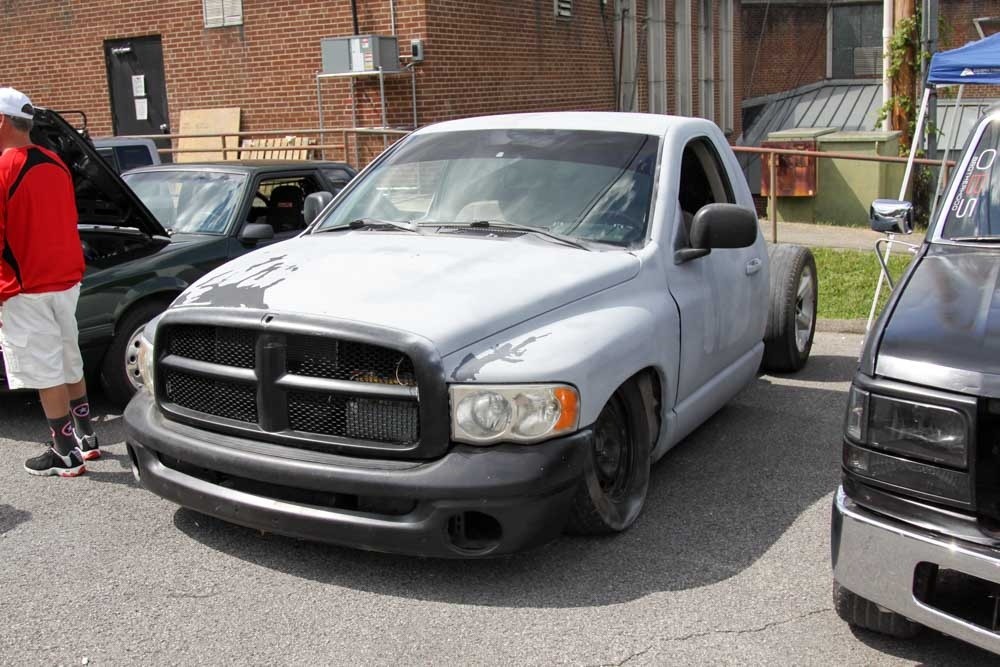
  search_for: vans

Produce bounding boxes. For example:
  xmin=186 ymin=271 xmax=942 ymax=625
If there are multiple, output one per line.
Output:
xmin=829 ymin=97 xmax=1000 ymax=667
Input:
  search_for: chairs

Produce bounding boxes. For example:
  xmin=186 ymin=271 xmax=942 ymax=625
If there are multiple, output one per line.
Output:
xmin=267 ymin=186 xmax=305 ymax=232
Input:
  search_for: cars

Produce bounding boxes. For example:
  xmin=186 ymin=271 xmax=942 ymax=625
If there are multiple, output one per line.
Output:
xmin=0 ymin=105 xmax=430 ymax=408
xmin=121 ymin=110 xmax=772 ymax=562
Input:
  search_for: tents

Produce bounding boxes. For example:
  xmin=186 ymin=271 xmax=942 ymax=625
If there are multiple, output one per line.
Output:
xmin=866 ymin=32 xmax=1000 ymax=330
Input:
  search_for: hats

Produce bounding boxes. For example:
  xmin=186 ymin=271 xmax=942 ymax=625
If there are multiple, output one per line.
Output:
xmin=0 ymin=87 xmax=35 ymax=119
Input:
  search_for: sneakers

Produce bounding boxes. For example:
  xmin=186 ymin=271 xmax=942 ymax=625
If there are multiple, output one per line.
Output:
xmin=24 ymin=447 xmax=85 ymax=477
xmin=72 ymin=427 xmax=102 ymax=459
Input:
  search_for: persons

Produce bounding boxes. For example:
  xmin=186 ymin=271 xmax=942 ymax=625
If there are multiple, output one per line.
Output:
xmin=0 ymin=88 xmax=101 ymax=477
